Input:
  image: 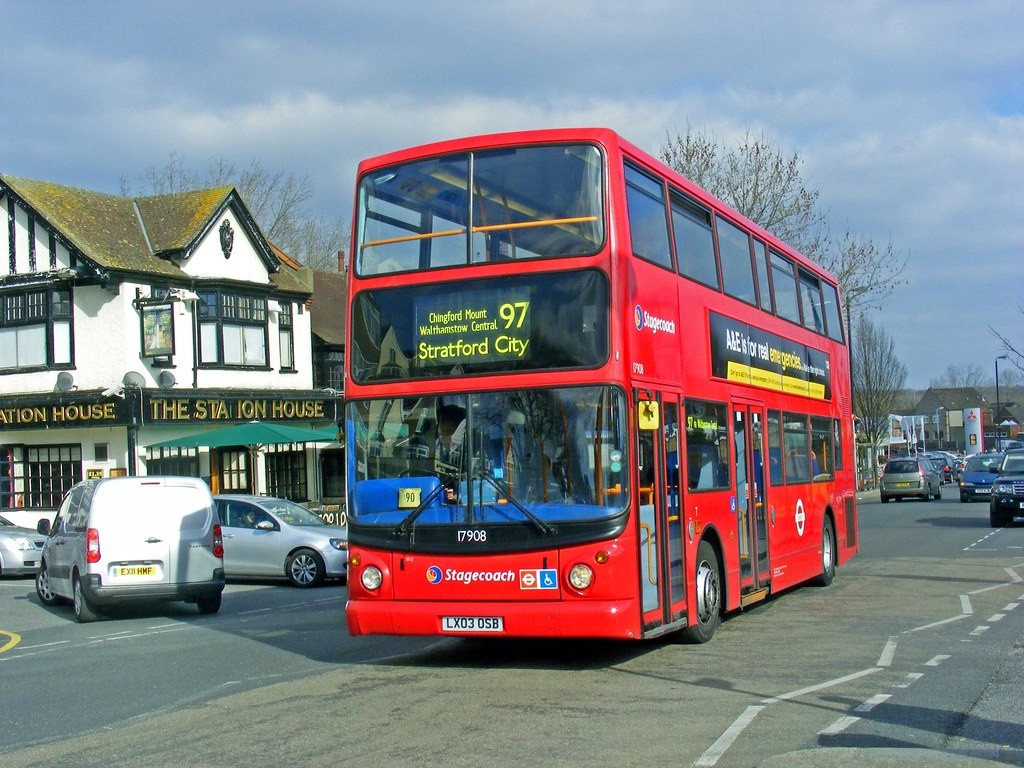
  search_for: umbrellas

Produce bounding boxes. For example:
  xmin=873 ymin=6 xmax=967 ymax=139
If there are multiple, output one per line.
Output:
xmin=313 ymin=422 xmax=408 ymax=478
xmin=144 ymin=421 xmax=336 ymax=497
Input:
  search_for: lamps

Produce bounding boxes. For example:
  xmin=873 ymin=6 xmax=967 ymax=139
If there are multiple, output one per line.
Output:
xmin=56 ymin=371 xmax=78 ymax=390
xmin=123 ymin=372 xmax=146 ymax=390
xmin=56 ymin=268 xmax=79 ymax=280
xmin=161 ymin=370 xmax=176 ymax=387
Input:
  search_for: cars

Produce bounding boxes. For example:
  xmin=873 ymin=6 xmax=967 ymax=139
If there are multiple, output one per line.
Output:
xmin=919 ymin=452 xmax=976 ymax=485
xmin=212 ymin=493 xmax=347 ymax=587
xmin=0 ymin=515 xmax=48 ymax=578
xmin=960 ymin=453 xmax=1005 ymax=503
xmin=879 ymin=458 xmax=942 ymax=503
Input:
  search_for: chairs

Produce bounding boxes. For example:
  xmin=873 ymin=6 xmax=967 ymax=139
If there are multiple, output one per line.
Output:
xmin=650 ymin=443 xmax=818 ymax=522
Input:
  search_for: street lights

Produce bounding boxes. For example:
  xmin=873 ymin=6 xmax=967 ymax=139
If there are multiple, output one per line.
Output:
xmin=995 ymin=356 xmax=1006 ymax=453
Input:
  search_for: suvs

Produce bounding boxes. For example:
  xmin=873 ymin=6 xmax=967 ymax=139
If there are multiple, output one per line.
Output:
xmin=990 ymin=449 xmax=1024 ymax=527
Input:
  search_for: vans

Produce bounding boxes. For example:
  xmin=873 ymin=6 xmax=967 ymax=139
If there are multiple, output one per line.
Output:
xmin=36 ymin=475 xmax=226 ymax=622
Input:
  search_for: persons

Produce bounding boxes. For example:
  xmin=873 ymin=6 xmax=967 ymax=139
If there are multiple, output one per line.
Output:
xmin=435 ymin=414 xmax=468 ymax=503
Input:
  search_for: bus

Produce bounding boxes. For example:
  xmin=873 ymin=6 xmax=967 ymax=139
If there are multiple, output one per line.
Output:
xmin=337 ymin=127 xmax=859 ymax=645
